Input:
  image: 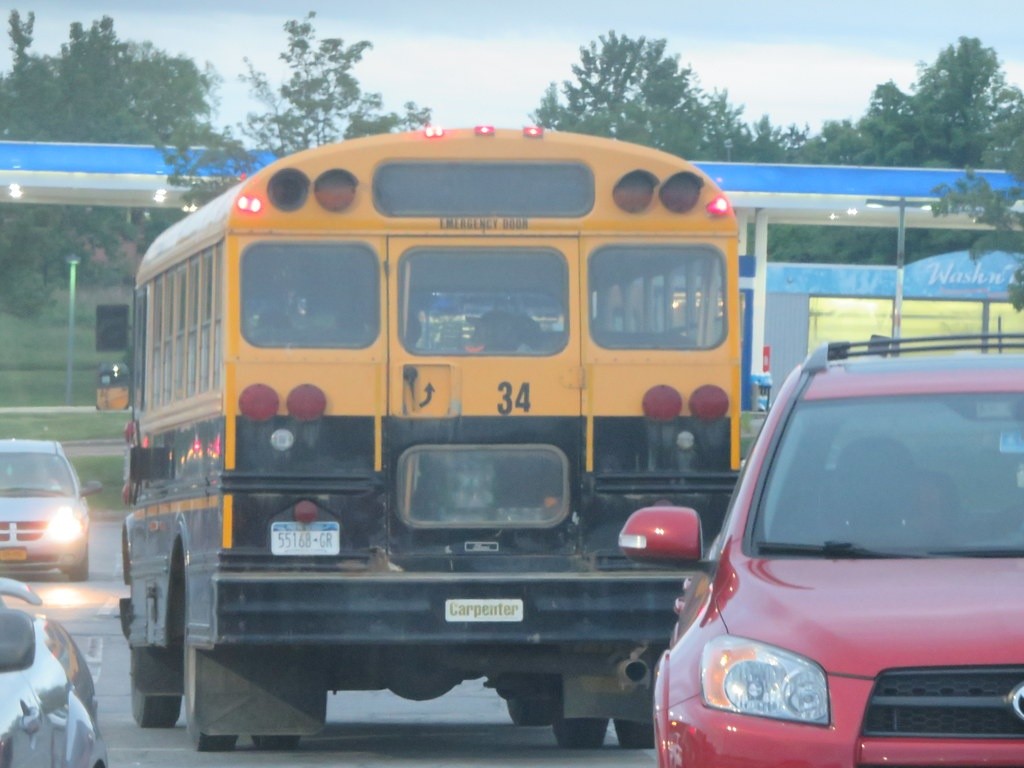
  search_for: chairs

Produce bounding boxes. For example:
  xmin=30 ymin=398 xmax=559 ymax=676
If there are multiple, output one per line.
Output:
xmin=835 ymin=440 xmax=938 ymax=547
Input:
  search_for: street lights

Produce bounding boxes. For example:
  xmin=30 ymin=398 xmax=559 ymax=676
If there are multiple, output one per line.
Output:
xmin=63 ymin=253 xmax=83 ymax=405
xmin=866 ymin=197 xmax=942 ymax=356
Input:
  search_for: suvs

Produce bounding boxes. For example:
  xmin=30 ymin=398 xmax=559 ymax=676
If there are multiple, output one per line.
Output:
xmin=615 ymin=331 xmax=1024 ymax=768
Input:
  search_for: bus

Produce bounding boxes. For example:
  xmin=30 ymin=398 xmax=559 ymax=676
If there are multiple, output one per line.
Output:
xmin=93 ymin=124 xmax=743 ymax=753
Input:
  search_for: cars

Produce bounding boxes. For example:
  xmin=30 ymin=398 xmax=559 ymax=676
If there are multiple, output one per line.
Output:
xmin=0 ymin=438 xmax=91 ymax=583
xmin=0 ymin=578 xmax=109 ymax=768
